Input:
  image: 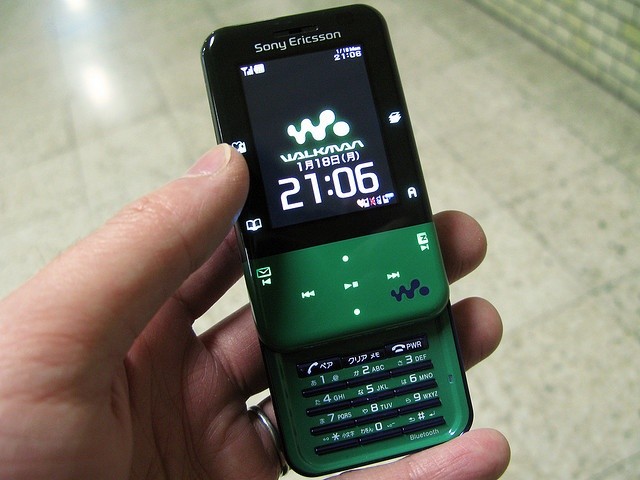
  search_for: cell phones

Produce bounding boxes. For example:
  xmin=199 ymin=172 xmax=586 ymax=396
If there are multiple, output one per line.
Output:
xmin=200 ymin=5 xmax=474 ymax=474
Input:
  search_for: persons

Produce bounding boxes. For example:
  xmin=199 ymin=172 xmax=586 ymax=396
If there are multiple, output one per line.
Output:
xmin=1 ymin=142 xmax=511 ymax=479
